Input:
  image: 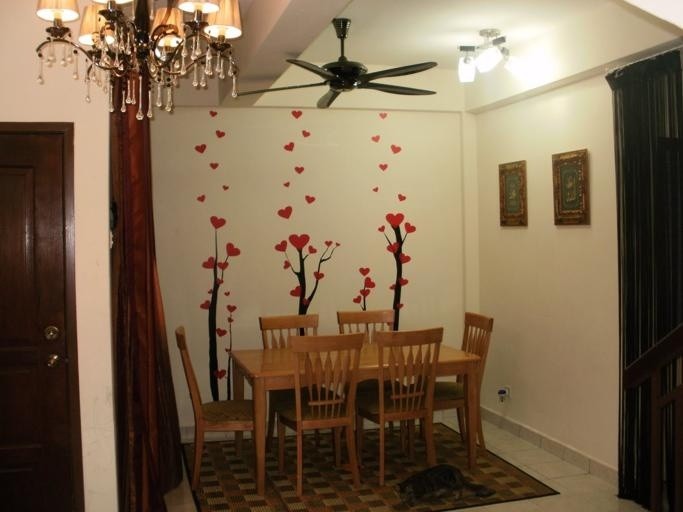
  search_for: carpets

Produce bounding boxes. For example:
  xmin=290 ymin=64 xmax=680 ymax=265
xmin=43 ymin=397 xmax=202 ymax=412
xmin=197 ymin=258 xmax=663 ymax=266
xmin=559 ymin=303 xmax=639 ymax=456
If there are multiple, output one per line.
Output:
xmin=173 ymin=422 xmax=566 ymax=512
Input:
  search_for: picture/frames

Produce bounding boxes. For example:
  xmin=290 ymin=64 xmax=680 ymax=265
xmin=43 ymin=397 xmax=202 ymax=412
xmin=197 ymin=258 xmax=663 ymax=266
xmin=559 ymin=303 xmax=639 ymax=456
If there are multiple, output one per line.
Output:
xmin=497 ymin=158 xmax=531 ymax=230
xmin=548 ymin=148 xmax=591 ymax=227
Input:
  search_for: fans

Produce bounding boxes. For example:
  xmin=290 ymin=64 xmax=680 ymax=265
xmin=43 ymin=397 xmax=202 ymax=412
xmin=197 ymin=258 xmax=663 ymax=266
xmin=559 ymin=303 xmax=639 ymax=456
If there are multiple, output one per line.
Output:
xmin=230 ymin=14 xmax=440 ymax=112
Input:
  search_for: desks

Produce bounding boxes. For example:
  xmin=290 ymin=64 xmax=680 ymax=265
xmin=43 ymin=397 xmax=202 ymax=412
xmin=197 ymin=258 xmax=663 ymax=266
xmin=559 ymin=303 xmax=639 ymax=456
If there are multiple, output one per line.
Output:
xmin=230 ymin=344 xmax=479 ymax=495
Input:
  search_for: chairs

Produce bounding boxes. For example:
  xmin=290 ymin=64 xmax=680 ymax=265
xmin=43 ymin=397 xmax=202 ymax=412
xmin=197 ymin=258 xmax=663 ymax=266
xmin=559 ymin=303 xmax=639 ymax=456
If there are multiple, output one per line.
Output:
xmin=337 ymin=309 xmax=408 ymax=459
xmin=276 ymin=333 xmax=365 ymax=498
xmin=407 ymin=311 xmax=494 ymax=460
xmin=258 ymin=314 xmax=320 ymax=455
xmin=356 ymin=327 xmax=444 ymax=487
xmin=174 ymin=326 xmax=265 ymax=493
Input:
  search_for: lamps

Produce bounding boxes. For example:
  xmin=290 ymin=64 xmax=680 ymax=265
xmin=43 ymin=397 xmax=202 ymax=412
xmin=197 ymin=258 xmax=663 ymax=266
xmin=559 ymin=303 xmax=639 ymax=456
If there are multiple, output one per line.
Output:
xmin=454 ymin=21 xmax=509 ymax=84
xmin=30 ymin=1 xmax=246 ymax=122
